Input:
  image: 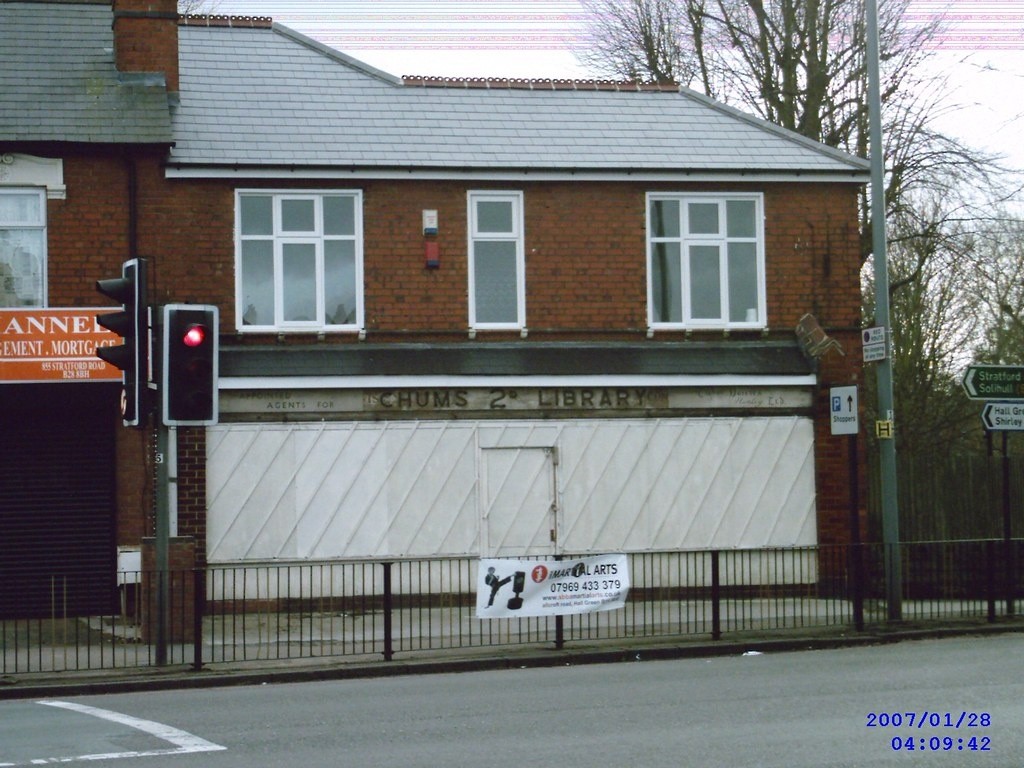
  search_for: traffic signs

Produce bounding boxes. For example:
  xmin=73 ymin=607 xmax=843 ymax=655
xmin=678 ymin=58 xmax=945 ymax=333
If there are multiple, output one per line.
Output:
xmin=981 ymin=403 xmax=1024 ymax=432
xmin=828 ymin=386 xmax=859 ymax=436
xmin=961 ymin=365 xmax=1024 ymax=402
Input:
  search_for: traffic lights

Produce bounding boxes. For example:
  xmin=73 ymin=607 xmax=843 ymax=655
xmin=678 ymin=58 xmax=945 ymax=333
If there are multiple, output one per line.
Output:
xmin=161 ymin=302 xmax=219 ymax=426
xmin=95 ymin=255 xmax=150 ymax=428
